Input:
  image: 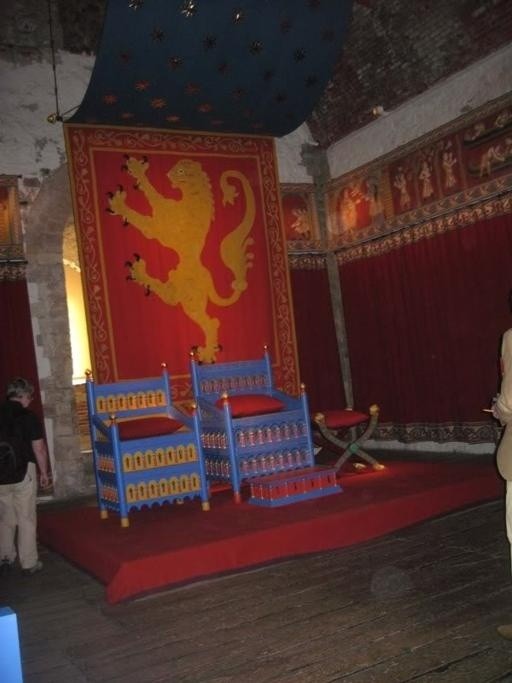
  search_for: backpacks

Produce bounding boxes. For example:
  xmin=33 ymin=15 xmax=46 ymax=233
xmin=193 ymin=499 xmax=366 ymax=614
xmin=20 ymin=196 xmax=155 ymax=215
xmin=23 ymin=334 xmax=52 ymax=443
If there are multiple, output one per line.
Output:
xmin=0 ymin=409 xmax=33 ymax=485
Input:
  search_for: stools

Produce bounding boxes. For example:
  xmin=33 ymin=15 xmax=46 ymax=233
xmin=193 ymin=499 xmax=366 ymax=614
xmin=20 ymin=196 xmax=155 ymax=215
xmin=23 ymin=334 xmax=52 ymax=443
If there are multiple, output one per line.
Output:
xmin=307 ymin=404 xmax=387 ymax=472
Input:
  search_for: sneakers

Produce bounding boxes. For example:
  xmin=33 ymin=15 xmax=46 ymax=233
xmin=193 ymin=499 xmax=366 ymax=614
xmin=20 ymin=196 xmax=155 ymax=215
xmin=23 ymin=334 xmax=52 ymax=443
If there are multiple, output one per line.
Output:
xmin=0 ymin=560 xmax=43 ymax=576
xmin=496 ymin=623 xmax=511 ymax=639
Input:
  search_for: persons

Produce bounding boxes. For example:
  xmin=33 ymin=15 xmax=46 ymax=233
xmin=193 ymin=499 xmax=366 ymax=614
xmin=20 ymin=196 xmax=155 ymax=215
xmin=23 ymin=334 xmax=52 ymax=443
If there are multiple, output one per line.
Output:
xmin=0 ymin=376 xmax=49 ymax=575
xmin=491 ymin=329 xmax=512 ymax=640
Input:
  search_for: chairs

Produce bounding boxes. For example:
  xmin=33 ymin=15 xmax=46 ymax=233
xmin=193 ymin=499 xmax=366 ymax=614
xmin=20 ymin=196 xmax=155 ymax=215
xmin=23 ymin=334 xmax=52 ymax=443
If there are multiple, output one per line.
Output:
xmin=86 ymin=363 xmax=210 ymax=527
xmin=189 ymin=345 xmax=315 ymax=504
